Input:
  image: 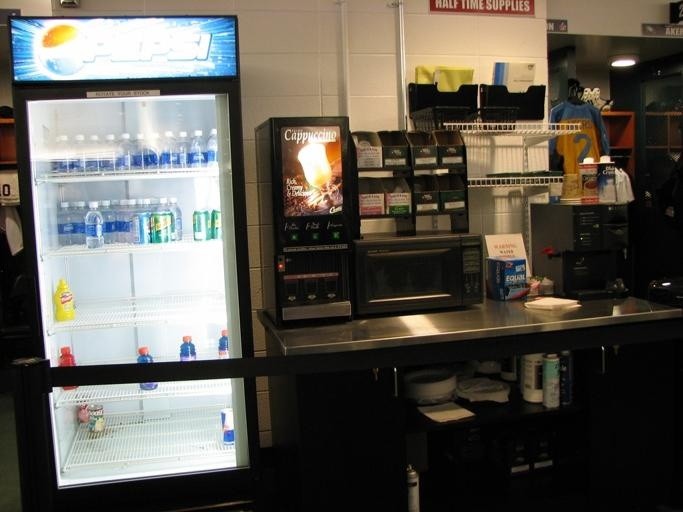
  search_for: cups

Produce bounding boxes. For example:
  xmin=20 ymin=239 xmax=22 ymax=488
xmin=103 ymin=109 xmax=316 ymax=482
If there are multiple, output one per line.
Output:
xmin=559 ymin=174 xmax=582 ymax=204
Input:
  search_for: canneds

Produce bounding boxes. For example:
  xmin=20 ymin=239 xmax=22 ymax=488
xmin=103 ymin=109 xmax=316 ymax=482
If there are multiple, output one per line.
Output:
xmin=210 ymin=210 xmax=222 ymax=239
xmin=131 ymin=211 xmax=152 ymax=245
xmin=598 ymin=162 xmax=616 ymax=203
xmin=75 ymin=396 xmax=90 ymax=427
xmin=578 ymin=162 xmax=599 ymax=203
xmin=193 ymin=208 xmax=211 ymax=241
xmin=87 ymin=405 xmax=105 ymax=432
xmin=150 ymin=210 xmax=175 ymax=243
xmin=221 ymin=408 xmax=235 ymax=445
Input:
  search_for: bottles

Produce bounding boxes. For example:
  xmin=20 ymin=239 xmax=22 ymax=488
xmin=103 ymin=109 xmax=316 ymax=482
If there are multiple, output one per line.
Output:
xmin=50 ymin=128 xmax=222 ymax=248
xmin=55 ymin=280 xmax=76 ymax=323
xmin=181 ymin=337 xmax=198 ymax=362
xmin=138 ymin=347 xmax=158 ymax=392
xmin=58 ymin=348 xmax=81 ymax=391
xmin=543 ymin=350 xmax=573 ymax=408
xmin=219 ymin=330 xmax=231 ymax=360
xmin=406 ymin=464 xmax=420 ymax=512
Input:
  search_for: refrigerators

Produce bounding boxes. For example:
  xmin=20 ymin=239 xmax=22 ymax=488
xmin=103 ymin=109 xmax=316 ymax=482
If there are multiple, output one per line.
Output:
xmin=8 ymin=15 xmax=262 ymax=512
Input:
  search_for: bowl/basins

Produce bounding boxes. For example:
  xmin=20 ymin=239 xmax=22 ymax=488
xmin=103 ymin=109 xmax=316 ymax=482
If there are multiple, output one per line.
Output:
xmin=410 ymin=370 xmax=457 ymax=405
